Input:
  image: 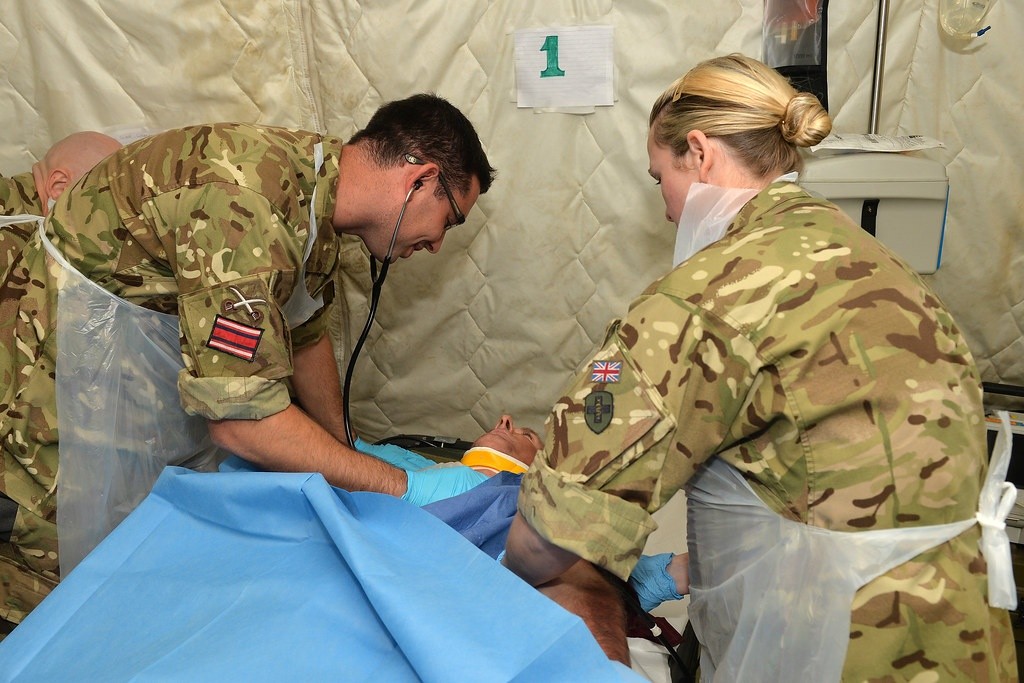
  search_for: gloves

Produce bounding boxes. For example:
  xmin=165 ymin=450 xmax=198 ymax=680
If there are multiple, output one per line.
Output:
xmin=398 ymin=464 xmax=489 ymax=507
xmin=354 ymin=435 xmax=438 ymax=474
xmin=629 ymin=553 xmax=684 ymax=611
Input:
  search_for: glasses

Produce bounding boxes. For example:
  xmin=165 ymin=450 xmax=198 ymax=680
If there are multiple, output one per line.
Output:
xmin=405 ymin=154 xmax=466 ymax=227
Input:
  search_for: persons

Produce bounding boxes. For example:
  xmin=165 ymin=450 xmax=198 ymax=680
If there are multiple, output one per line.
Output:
xmin=1 ymin=130 xmax=126 ymax=282
xmin=1 ymin=94 xmax=500 ymax=564
xmin=420 ymin=414 xmax=631 ymax=667
xmin=503 ymin=51 xmax=1019 ymax=683
